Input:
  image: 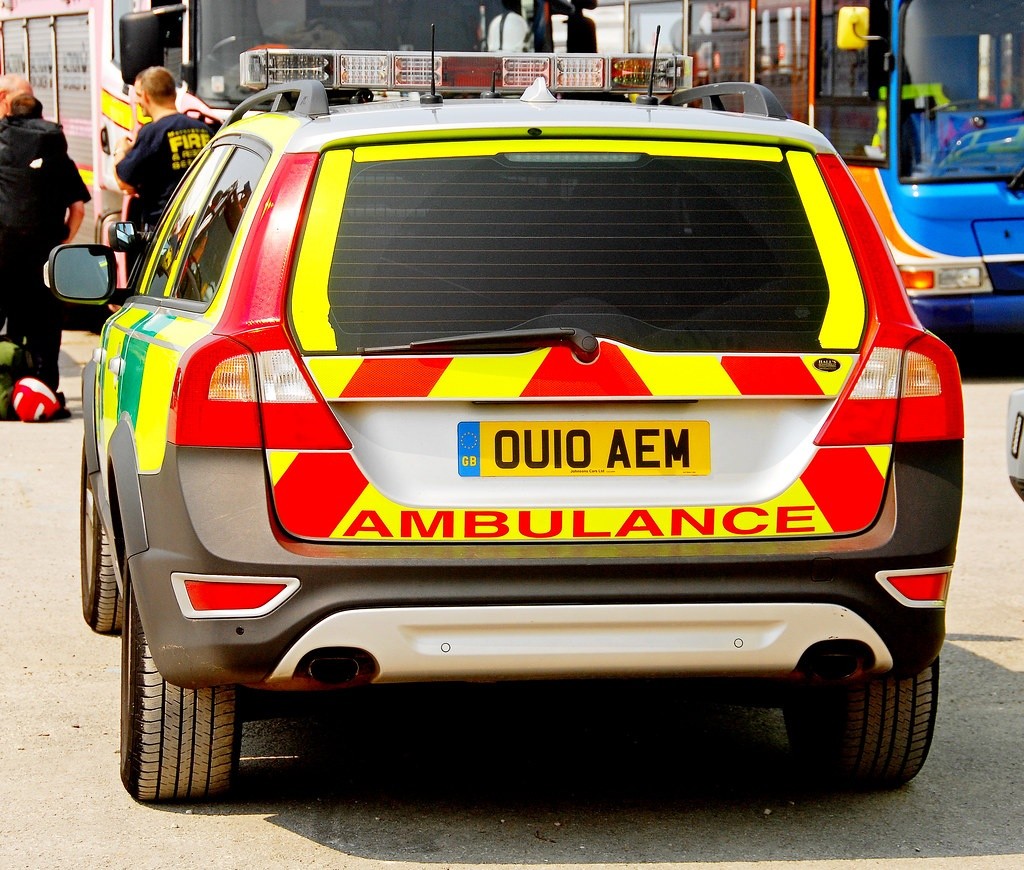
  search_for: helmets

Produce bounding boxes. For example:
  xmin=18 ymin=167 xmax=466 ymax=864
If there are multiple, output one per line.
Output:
xmin=12 ymin=377 xmax=62 ymax=422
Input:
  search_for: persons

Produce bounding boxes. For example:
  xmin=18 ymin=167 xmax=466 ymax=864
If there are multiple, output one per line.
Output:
xmin=118 ymin=66 xmax=162 ymax=282
xmin=112 ymin=61 xmax=218 ymax=234
xmin=0 ymin=91 xmax=94 ymax=422
xmin=0 ymin=68 xmax=47 ymax=350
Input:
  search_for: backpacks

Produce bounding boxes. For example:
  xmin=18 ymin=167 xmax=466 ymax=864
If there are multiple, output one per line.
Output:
xmin=0 ymin=334 xmax=35 ymax=422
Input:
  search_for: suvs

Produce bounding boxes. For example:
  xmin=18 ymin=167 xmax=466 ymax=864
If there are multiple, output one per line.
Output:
xmin=40 ymin=49 xmax=964 ymax=806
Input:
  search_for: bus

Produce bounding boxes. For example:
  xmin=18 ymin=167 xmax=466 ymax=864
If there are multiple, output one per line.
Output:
xmin=0 ymin=0 xmax=599 ymax=334
xmin=484 ymin=2 xmax=1024 ymax=376
